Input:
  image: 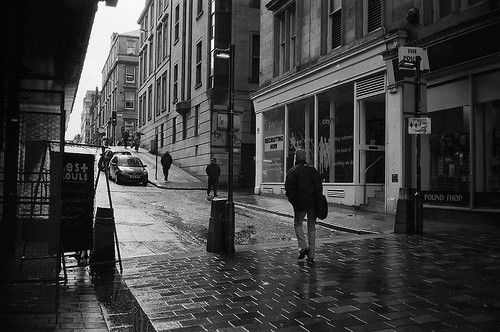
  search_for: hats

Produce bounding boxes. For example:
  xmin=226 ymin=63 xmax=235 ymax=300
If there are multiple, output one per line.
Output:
xmin=296 ymin=149 xmax=306 ymax=161
xmin=211 ymin=157 xmax=217 ymax=162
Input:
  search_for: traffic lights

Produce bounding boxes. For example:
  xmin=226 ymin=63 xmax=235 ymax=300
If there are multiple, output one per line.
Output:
xmin=112 ymin=110 xmax=117 ymax=127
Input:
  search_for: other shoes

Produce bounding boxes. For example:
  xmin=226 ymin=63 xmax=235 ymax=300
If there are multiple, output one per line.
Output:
xmin=306 ymin=257 xmax=315 ymax=262
xmin=297 ymin=248 xmax=309 ymax=260
xmin=214 ymin=195 xmax=218 ymax=197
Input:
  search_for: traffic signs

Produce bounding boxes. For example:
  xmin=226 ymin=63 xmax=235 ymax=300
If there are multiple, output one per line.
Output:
xmin=408 ymin=118 xmax=432 ymax=135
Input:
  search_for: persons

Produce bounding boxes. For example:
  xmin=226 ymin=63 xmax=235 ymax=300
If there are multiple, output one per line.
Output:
xmin=123 ymin=130 xmax=141 ymax=152
xmin=206 ymin=158 xmax=221 ymax=197
xmin=285 ymin=149 xmax=323 ymax=262
xmin=161 ymin=151 xmax=172 ymax=180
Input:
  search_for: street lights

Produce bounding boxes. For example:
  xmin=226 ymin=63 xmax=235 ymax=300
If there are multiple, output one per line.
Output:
xmin=214 ymin=44 xmax=235 ymax=256
xmin=399 ymin=56 xmax=424 ymax=236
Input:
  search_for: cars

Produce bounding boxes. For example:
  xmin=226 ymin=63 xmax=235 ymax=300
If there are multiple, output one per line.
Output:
xmin=107 ymin=155 xmax=148 ymax=186
xmin=98 ymin=149 xmax=132 ymax=172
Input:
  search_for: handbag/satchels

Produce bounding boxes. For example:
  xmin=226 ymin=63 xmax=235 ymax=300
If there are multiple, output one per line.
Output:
xmin=316 ymin=194 xmax=328 ymax=220
